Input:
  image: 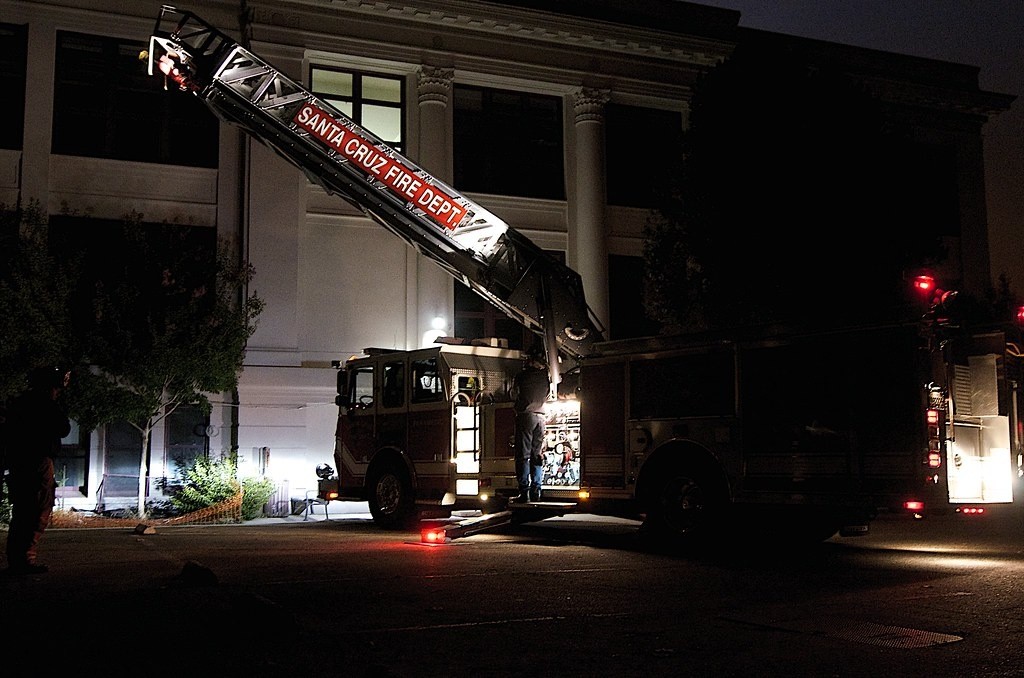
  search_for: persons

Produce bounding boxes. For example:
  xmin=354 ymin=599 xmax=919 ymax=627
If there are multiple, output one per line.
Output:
xmin=0 ymin=363 xmax=73 ymax=575
xmin=508 ymin=345 xmax=550 ymax=503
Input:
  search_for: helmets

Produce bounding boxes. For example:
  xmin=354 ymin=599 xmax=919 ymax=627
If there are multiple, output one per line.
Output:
xmin=525 ymin=346 xmax=546 ymax=365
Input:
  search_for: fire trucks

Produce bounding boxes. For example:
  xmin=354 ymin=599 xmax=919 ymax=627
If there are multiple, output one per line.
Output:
xmin=139 ymin=3 xmax=1024 ymax=553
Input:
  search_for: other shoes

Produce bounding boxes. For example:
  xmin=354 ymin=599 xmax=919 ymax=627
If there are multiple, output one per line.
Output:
xmin=531 ymin=493 xmax=541 ymax=501
xmin=509 ymin=490 xmax=530 ymax=503
